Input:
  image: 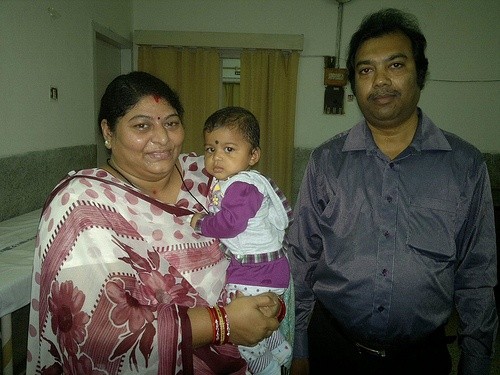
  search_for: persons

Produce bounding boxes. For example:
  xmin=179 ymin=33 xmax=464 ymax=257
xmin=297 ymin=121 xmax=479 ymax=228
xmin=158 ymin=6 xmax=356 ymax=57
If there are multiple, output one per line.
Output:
xmin=189 ymin=106 xmax=293 ymax=375
xmin=291 ymin=8 xmax=497 ymax=375
xmin=25 ymin=70 xmax=288 ymax=375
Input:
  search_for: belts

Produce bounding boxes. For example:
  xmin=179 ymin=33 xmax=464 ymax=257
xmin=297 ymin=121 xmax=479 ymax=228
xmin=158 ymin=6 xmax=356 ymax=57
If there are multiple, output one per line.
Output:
xmin=325 ymin=313 xmax=458 ymax=363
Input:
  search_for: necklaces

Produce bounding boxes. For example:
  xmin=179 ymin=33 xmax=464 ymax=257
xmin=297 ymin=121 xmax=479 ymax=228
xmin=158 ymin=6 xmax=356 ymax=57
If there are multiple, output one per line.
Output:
xmin=106 ymin=156 xmax=210 ymax=217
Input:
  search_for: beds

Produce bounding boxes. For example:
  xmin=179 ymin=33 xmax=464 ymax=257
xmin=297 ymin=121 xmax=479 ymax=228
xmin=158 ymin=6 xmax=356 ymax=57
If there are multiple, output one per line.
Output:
xmin=0 ymin=206 xmax=46 ymax=375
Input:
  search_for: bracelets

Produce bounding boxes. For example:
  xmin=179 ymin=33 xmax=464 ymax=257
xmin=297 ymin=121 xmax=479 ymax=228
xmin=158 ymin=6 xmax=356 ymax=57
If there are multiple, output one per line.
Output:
xmin=272 ymin=294 xmax=285 ymax=325
xmin=207 ymin=307 xmax=232 ymax=346
